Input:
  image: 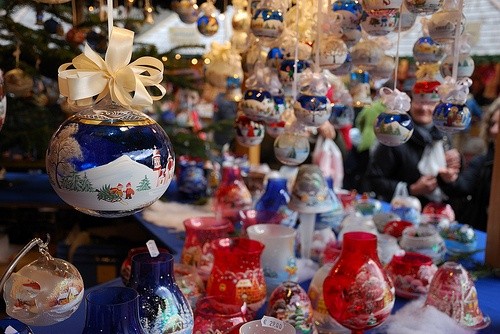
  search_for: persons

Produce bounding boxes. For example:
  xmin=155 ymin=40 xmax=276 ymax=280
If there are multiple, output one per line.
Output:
xmin=164 ymin=70 xmax=500 ymax=234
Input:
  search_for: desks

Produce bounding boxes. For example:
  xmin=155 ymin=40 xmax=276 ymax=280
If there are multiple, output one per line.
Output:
xmin=0 ymin=156 xmax=500 ymax=334
